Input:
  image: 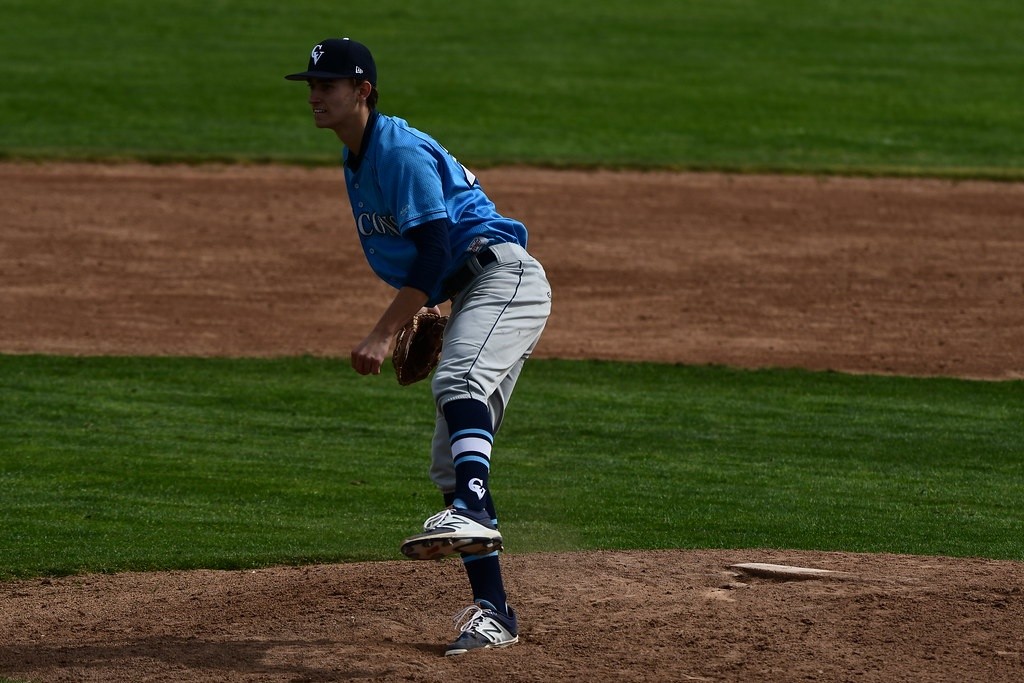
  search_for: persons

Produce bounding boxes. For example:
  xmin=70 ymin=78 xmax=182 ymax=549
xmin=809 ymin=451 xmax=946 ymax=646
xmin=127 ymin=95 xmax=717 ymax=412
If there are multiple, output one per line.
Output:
xmin=286 ymin=39 xmax=552 ymax=657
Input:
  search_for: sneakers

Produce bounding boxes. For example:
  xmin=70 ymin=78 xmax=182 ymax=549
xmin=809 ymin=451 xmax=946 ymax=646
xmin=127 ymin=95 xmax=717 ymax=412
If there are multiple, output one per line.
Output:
xmin=444 ymin=600 xmax=520 ymax=656
xmin=399 ymin=504 xmax=504 ymax=561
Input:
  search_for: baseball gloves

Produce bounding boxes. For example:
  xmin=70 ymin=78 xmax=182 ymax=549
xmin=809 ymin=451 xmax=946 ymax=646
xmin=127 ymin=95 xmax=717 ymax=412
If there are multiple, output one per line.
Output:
xmin=392 ymin=312 xmax=451 ymax=386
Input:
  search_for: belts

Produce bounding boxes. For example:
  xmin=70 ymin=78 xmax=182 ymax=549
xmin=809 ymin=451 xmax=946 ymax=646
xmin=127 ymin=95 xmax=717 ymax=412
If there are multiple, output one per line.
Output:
xmin=443 ymin=249 xmax=497 ymax=298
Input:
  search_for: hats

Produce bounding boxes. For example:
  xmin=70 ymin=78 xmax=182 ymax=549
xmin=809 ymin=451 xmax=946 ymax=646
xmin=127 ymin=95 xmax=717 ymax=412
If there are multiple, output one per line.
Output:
xmin=283 ymin=36 xmax=378 ymax=85
